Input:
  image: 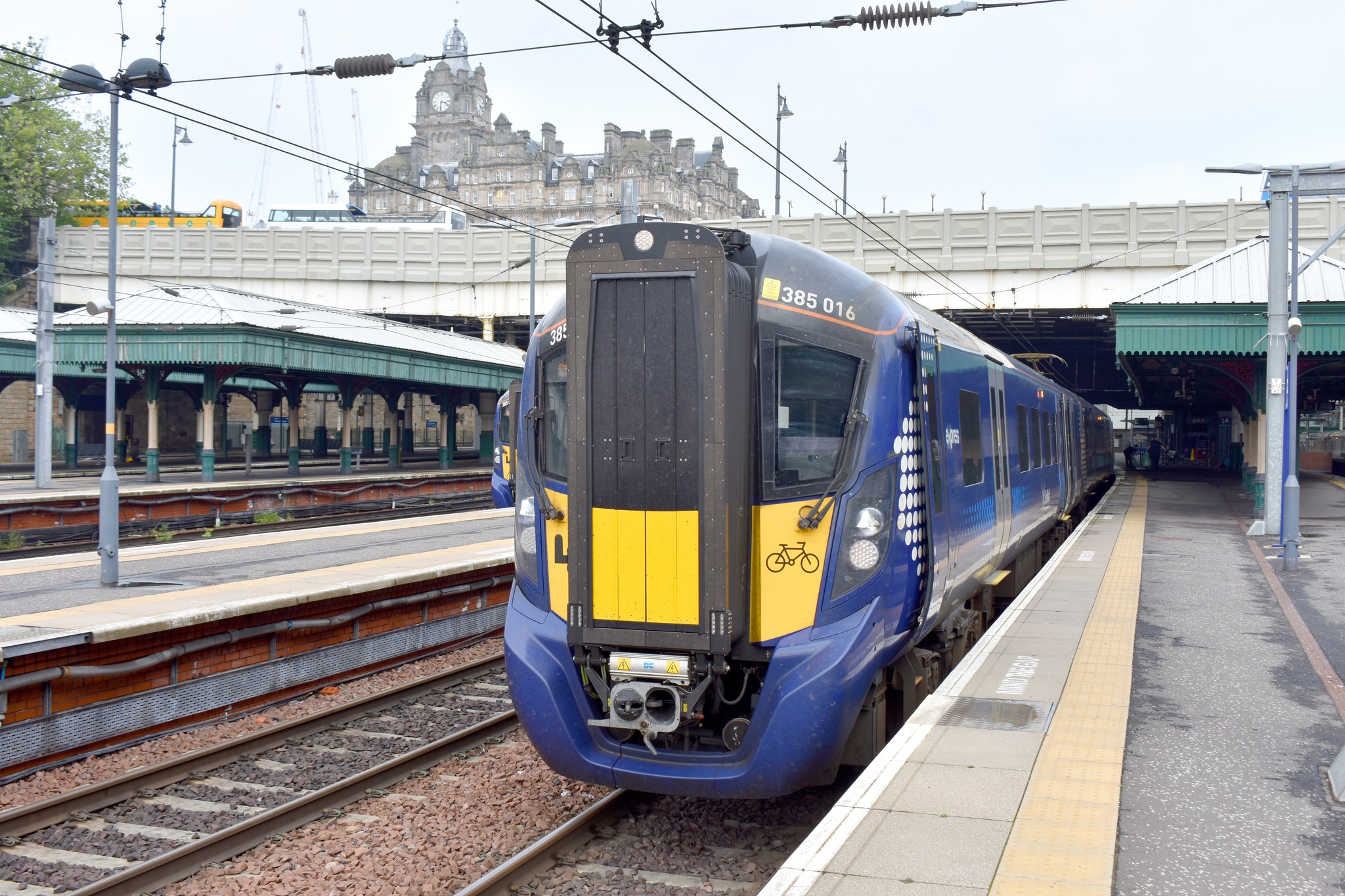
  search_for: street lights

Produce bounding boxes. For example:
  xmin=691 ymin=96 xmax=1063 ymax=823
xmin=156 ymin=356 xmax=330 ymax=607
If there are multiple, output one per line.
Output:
xmin=697 ymin=202 xmax=702 ymax=217
xmin=741 ymin=200 xmax=747 ymax=218
xmin=881 ymin=193 xmax=888 ymax=213
xmin=929 ymin=192 xmax=936 ymax=211
xmin=1202 ymin=161 xmax=1345 ymax=568
xmin=170 ymin=115 xmax=193 ymax=231
xmin=653 ymin=204 xmax=659 ymax=215
xmin=774 ymin=84 xmax=794 ymax=214
xmin=833 ymin=138 xmax=848 ymax=215
xmin=980 ymin=190 xmax=986 ymax=210
xmin=56 ymin=58 xmax=175 ymax=585
xmin=786 ymin=199 xmax=793 ymax=217
xmin=344 ymin=161 xmax=361 ymax=206
xmin=834 ymin=196 xmax=840 ymax=216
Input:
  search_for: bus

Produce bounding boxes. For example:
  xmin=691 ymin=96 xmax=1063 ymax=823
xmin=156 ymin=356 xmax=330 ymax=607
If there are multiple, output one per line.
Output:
xmin=58 ymin=194 xmax=243 ymax=229
xmin=267 ymin=200 xmax=468 ymax=231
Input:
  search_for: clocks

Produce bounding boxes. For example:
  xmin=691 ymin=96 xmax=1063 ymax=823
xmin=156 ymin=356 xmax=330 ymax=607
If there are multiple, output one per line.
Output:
xmin=432 ymin=91 xmax=451 ymax=112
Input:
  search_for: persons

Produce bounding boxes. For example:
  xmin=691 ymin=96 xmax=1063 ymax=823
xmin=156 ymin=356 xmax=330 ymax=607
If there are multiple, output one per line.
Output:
xmin=1123 ymin=447 xmax=1138 ymax=471
xmin=1133 ymin=441 xmax=1138 ymax=457
xmin=1144 ymin=437 xmax=1168 ymax=473
xmin=1126 ymin=440 xmax=1131 ymax=448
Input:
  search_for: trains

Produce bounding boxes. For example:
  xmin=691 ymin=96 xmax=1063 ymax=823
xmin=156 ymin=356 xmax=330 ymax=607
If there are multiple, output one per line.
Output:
xmin=491 ymin=208 xmax=1114 ymax=797
